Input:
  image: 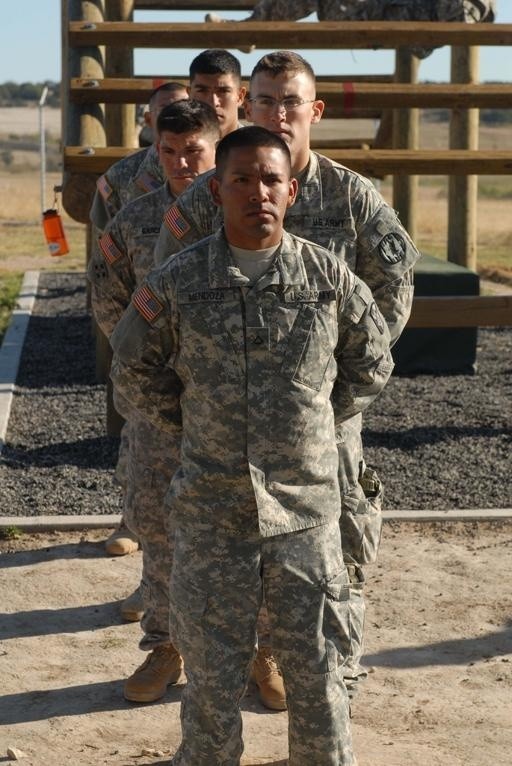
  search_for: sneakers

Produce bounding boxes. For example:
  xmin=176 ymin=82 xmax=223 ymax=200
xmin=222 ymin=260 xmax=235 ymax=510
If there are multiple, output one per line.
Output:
xmin=124 ymin=644 xmax=182 ymax=703
xmin=120 ymin=587 xmax=144 ymax=621
xmin=205 ymin=13 xmax=257 ymax=53
xmin=250 ymin=647 xmax=288 ymax=710
xmin=106 ymin=521 xmax=139 ymax=555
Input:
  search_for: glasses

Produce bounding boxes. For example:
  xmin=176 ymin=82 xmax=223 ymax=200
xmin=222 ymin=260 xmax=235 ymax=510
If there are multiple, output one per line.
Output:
xmin=245 ymin=95 xmax=313 ymax=111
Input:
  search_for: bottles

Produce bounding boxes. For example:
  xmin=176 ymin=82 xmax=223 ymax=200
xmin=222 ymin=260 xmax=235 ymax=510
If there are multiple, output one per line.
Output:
xmin=359 ymin=478 xmax=376 ymax=498
xmin=41 ymin=209 xmax=69 ymax=257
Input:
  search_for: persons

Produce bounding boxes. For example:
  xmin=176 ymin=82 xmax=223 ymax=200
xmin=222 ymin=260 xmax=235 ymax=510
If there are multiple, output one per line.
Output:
xmin=156 ymin=51 xmax=423 ymax=718
xmin=109 ymin=123 xmax=396 ymax=765
xmin=83 ymin=79 xmax=194 ymax=561
xmin=203 ymin=0 xmax=496 ymax=55
xmin=98 ymin=99 xmax=310 ymax=714
xmin=116 ymin=49 xmax=248 ymax=625
